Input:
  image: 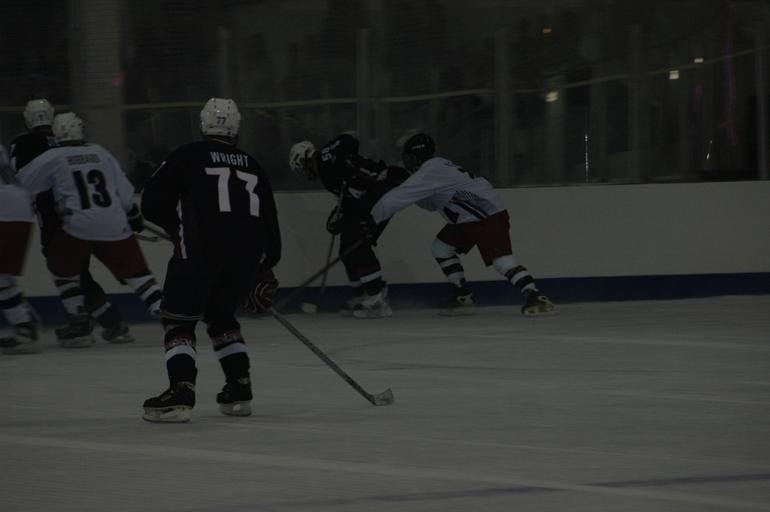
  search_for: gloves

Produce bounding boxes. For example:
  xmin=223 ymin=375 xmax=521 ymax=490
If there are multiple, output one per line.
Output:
xmin=127 ymin=203 xmax=145 ymax=233
xmin=325 ymin=206 xmax=346 ymax=234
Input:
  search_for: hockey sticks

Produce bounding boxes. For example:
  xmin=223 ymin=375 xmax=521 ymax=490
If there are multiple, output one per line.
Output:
xmin=142 ymin=219 xmax=394 ymax=405
xmin=302 ymin=179 xmax=349 ymax=313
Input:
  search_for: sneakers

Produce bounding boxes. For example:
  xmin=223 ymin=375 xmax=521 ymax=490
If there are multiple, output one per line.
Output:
xmin=353 ymin=285 xmax=385 ymax=313
xmin=55 ymin=316 xmax=91 ymax=338
xmin=1 ymin=322 xmax=36 ymax=348
xmin=217 ymin=372 xmax=252 ymax=403
xmin=102 ymin=319 xmax=129 ymax=341
xmin=438 ymin=284 xmax=477 ymax=309
xmin=143 ymin=378 xmax=195 ymax=407
xmin=522 ymin=289 xmax=549 ymax=313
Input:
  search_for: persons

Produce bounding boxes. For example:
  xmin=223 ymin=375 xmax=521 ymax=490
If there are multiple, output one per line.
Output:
xmin=288 ymin=132 xmax=413 ymax=318
xmin=357 ymin=132 xmax=557 ymax=316
xmin=0 ymin=98 xmax=283 ymax=423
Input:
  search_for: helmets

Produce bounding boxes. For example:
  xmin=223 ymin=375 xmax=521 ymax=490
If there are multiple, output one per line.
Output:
xmin=289 ymin=141 xmax=318 ymax=173
xmin=22 ymin=98 xmax=56 ymax=129
xmin=52 ymin=112 xmax=83 ymax=144
xmin=402 ymin=134 xmax=436 ymax=172
xmin=200 ymin=97 xmax=242 ymax=139
